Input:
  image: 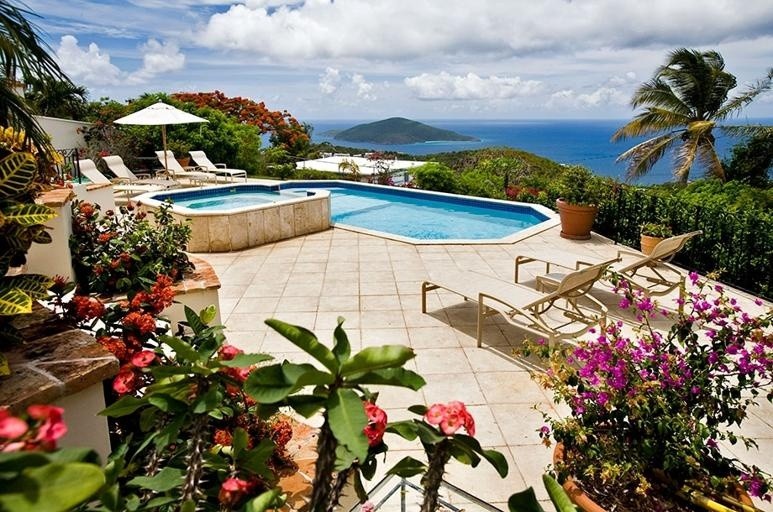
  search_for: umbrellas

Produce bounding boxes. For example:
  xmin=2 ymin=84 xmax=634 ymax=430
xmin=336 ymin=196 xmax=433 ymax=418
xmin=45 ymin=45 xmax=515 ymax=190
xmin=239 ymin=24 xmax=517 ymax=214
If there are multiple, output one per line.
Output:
xmin=112 ymin=100 xmax=210 ymax=188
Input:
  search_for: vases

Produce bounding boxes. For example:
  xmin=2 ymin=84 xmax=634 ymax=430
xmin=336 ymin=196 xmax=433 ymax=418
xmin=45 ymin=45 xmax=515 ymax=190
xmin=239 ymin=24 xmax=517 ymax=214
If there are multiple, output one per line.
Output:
xmin=552 ymin=434 xmax=755 ymax=512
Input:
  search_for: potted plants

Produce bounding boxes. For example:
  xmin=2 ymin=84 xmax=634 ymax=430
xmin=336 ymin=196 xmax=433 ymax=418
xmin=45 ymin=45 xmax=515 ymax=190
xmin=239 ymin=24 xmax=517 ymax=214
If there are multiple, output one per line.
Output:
xmin=637 ymin=221 xmax=675 ymax=258
xmin=554 ymin=164 xmax=605 ymax=242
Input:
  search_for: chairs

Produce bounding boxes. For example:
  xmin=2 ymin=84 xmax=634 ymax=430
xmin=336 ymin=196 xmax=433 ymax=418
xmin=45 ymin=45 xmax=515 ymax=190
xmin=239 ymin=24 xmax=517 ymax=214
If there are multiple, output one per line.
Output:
xmin=72 ymin=154 xmax=182 ymax=202
xmin=153 ymin=149 xmax=249 ymax=187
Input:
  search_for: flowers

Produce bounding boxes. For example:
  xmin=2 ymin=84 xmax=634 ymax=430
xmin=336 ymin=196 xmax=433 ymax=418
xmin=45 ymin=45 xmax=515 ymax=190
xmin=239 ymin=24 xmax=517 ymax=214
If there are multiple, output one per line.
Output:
xmin=512 ymin=269 xmax=773 ymax=498
xmin=311 ymin=396 xmax=507 ymax=512
